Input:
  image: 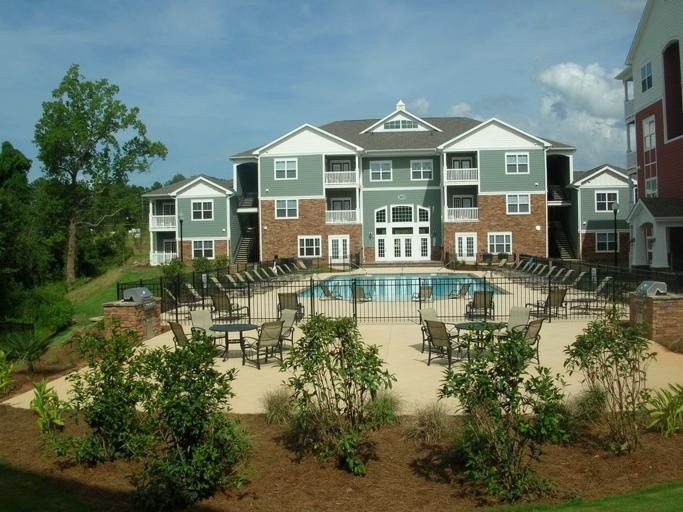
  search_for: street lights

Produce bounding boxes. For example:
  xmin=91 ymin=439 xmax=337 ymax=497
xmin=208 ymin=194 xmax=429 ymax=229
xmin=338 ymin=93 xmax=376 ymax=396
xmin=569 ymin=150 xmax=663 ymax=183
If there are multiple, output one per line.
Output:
xmin=179 ymin=211 xmax=184 ymax=262
xmin=611 ymin=200 xmax=618 ymax=317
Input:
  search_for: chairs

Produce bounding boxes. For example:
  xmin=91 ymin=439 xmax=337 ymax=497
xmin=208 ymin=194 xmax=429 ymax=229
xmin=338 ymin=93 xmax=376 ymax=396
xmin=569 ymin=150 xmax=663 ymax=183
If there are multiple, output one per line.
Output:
xmin=164 ymin=261 xmax=313 ymax=314
xmin=318 ymin=280 xmax=372 ymax=302
xmin=168 ymin=291 xmax=305 ymax=373
xmin=410 ymin=283 xmax=567 ymax=371
xmin=501 ymin=260 xmax=614 ymax=312
